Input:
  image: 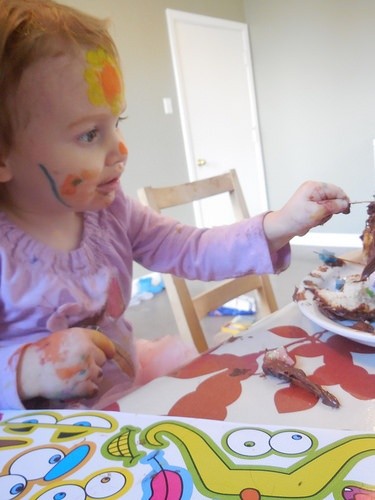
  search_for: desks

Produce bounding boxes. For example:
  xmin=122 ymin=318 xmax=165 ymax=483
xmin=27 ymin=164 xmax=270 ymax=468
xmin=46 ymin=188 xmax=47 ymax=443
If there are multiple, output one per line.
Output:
xmin=100 ymin=299 xmax=374 ymax=432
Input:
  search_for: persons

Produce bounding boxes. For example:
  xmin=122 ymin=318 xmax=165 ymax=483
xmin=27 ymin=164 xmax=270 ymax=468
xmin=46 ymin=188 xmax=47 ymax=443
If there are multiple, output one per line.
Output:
xmin=0 ymin=0 xmax=352 ymax=410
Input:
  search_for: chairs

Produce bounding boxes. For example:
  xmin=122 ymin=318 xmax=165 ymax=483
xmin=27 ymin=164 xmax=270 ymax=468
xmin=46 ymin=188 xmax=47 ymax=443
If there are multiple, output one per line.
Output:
xmin=137 ymin=169 xmax=278 ymax=354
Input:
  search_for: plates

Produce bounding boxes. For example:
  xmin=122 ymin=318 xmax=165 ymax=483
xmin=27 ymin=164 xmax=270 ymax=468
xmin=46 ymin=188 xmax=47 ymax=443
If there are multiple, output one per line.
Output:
xmin=297 ymin=250 xmax=375 ymax=347
